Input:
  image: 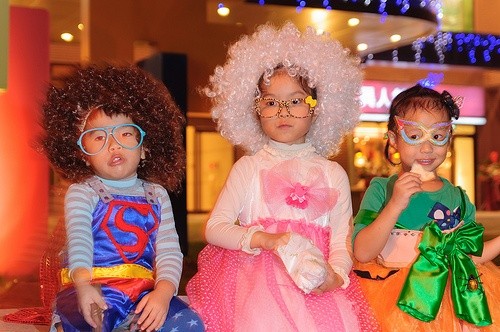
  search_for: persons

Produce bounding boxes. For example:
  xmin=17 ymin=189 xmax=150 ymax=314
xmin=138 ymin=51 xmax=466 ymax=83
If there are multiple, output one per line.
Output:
xmin=41 ymin=63 xmax=205 ymax=332
xmin=351 ymin=84 xmax=500 ymax=332
xmin=186 ymin=22 xmax=380 ymax=332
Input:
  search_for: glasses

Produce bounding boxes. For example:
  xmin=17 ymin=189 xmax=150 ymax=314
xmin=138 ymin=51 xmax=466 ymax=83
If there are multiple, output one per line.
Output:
xmin=253 ymin=95 xmax=316 ymax=120
xmin=76 ymin=124 xmax=146 ymax=156
